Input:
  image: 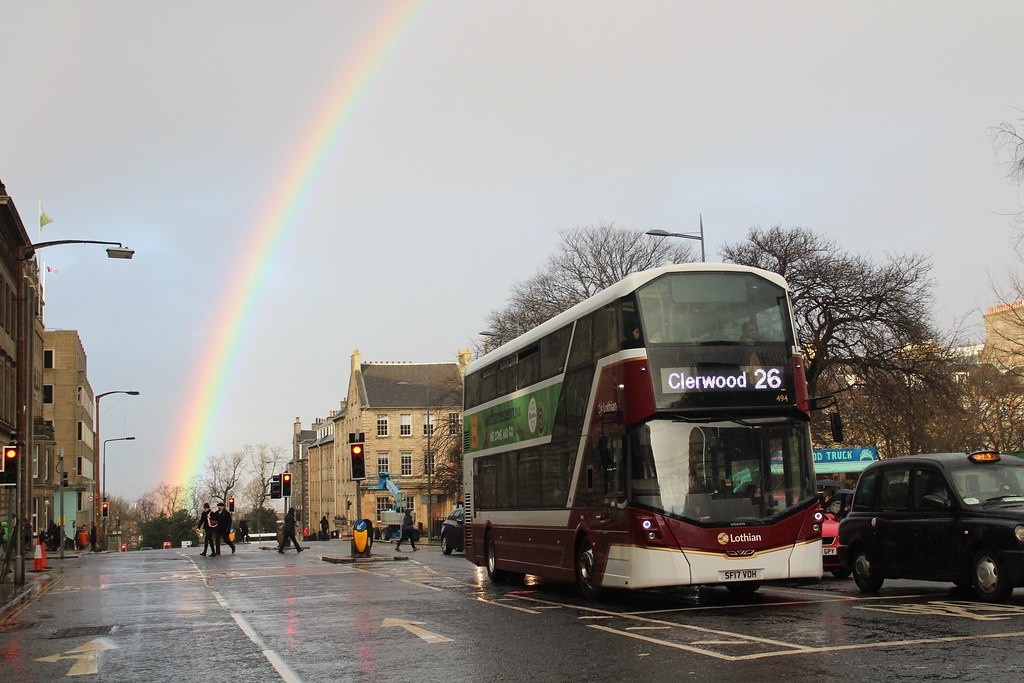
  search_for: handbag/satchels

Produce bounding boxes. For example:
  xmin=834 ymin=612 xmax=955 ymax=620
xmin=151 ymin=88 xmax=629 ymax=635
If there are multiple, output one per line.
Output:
xmin=207 ymin=512 xmax=218 ymax=527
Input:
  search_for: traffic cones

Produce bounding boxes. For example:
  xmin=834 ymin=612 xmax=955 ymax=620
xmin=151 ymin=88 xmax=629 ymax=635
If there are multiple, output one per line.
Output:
xmin=29 ymin=536 xmax=50 ymax=572
xmin=41 ymin=541 xmax=53 ymax=569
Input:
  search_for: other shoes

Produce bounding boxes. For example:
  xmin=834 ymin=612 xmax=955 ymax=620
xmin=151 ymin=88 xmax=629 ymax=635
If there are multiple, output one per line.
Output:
xmin=200 ymin=552 xmax=206 ymax=556
xmin=232 ymin=546 xmax=235 ymax=553
xmin=298 ymin=549 xmax=303 ymax=552
xmin=278 ymin=551 xmax=284 ymax=553
xmin=209 ymin=553 xmax=216 ymax=557
xmin=215 ymin=553 xmax=220 ymax=555
xmin=414 ymin=548 xmax=420 ymax=551
xmin=395 ymin=547 xmax=402 ymax=551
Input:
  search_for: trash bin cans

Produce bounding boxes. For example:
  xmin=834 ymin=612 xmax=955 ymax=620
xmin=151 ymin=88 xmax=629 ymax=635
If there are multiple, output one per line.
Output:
xmin=331 ymin=530 xmax=339 ymax=539
xmin=432 ymin=517 xmax=445 ymax=538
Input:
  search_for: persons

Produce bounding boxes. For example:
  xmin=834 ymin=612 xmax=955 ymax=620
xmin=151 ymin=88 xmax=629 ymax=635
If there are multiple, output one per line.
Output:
xmin=933 ymin=472 xmax=965 ymax=501
xmin=0 ymin=514 xmax=32 ymax=552
xmin=48 ymin=519 xmax=60 ymax=552
xmin=624 ymin=321 xmax=662 ymax=343
xmin=739 ymin=321 xmax=768 ymax=342
xmin=236 ymin=521 xmax=250 ymax=544
xmin=395 ymin=509 xmax=420 ymax=552
xmin=77 ymin=525 xmax=90 ymax=550
xmin=195 ymin=503 xmax=216 ymax=557
xmin=91 ymin=523 xmax=97 ymax=551
xmin=320 ymin=516 xmax=329 ymax=540
xmin=39 ymin=531 xmax=47 ymax=544
xmin=214 ymin=503 xmax=235 ymax=554
xmin=702 ymin=323 xmax=730 ymax=341
xmin=278 ymin=508 xmax=303 ymax=554
xmin=820 ymin=486 xmax=835 ymax=509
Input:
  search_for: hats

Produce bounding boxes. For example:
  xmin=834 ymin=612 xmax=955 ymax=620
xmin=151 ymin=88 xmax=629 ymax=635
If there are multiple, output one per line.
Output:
xmin=204 ymin=503 xmax=209 ymax=508
xmin=217 ymin=503 xmax=224 ymax=506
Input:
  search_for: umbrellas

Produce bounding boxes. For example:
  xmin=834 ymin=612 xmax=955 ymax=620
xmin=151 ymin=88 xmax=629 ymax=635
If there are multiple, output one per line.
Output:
xmin=817 ymin=478 xmax=842 ymax=486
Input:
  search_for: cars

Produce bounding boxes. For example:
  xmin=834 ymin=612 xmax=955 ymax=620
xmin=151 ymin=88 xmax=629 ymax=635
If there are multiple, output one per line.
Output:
xmin=441 ymin=507 xmax=464 ymax=556
xmin=838 ymin=449 xmax=1024 ymax=606
xmin=756 ymin=492 xmax=851 ymax=579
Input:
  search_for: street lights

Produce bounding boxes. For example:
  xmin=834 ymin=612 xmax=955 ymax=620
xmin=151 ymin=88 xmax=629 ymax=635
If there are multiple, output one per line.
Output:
xmin=94 ymin=390 xmax=140 ymax=549
xmin=396 ymin=372 xmax=432 ymax=543
xmin=15 ymin=239 xmax=135 ymax=586
xmin=102 ymin=437 xmax=135 ymax=551
xmin=646 ymin=212 xmax=706 ymax=261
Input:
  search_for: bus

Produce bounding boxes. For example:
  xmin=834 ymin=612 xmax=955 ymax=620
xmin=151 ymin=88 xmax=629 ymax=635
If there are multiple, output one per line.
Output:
xmin=463 ymin=262 xmax=843 ymax=605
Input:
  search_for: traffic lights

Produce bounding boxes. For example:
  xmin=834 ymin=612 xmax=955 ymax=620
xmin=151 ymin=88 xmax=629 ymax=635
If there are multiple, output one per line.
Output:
xmin=282 ymin=473 xmax=292 ymax=497
xmin=102 ymin=502 xmax=109 ymax=518
xmin=387 ymin=504 xmax=393 ymax=511
xmin=0 ymin=445 xmax=17 ymax=486
xmin=229 ymin=497 xmax=235 ymax=513
xmin=270 ymin=473 xmax=282 ymax=499
xmin=350 ymin=442 xmax=367 ymax=481
